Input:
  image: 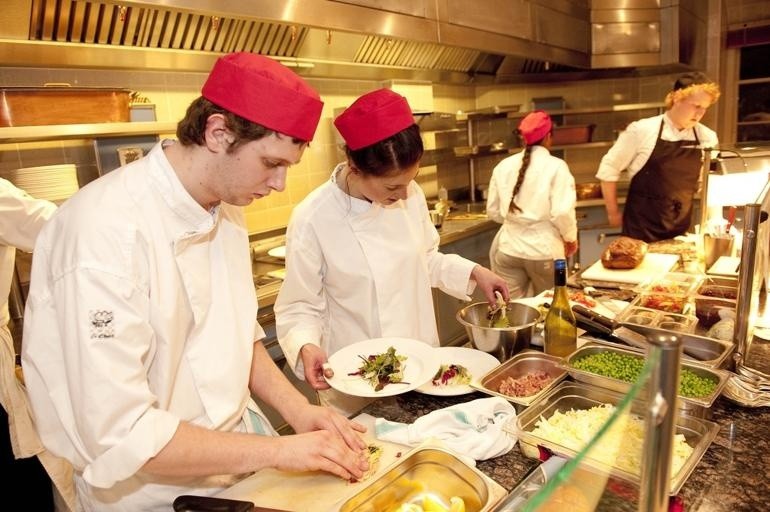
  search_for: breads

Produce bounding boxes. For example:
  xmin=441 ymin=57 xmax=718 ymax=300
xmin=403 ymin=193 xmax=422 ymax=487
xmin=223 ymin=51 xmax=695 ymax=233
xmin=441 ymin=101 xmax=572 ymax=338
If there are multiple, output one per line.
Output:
xmin=601 ymin=236 xmax=649 ymax=269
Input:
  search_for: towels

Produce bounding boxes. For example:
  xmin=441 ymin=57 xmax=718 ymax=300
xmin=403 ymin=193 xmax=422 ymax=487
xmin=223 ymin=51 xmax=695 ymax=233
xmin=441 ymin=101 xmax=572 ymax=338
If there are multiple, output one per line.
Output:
xmin=375 ymin=396 xmax=519 ymax=471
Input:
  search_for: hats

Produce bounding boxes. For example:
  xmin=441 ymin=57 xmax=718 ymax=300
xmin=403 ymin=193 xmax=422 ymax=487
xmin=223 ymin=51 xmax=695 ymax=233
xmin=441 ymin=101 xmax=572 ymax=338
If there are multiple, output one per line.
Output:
xmin=519 ymin=110 xmax=552 ymax=146
xmin=333 ymin=89 xmax=415 ymax=151
xmin=672 ymin=72 xmax=713 ymax=91
xmin=201 ymin=55 xmax=323 ymax=142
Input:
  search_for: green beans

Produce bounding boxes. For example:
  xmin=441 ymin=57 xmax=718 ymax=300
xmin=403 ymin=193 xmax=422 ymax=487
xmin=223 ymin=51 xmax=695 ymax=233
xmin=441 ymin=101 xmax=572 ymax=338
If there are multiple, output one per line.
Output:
xmin=571 ymin=350 xmax=718 ymax=399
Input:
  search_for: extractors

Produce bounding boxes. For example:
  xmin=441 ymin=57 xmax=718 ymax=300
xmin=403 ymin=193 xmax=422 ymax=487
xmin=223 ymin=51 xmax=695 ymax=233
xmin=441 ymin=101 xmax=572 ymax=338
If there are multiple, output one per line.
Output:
xmin=26 ymin=1 xmax=591 ymax=78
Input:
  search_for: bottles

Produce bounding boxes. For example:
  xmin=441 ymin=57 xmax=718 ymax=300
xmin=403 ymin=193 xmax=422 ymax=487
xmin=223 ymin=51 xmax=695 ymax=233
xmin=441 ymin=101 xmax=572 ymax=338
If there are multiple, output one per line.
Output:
xmin=437 ymin=185 xmax=449 ymax=205
xmin=543 ymin=259 xmax=577 ymax=354
xmin=435 ymin=199 xmax=449 ymax=218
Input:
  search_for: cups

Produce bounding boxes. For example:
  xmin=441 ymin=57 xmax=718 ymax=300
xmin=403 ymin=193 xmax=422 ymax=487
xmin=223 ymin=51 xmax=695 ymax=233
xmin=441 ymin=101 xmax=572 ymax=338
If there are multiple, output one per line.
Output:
xmin=704 ymin=233 xmax=734 ymax=272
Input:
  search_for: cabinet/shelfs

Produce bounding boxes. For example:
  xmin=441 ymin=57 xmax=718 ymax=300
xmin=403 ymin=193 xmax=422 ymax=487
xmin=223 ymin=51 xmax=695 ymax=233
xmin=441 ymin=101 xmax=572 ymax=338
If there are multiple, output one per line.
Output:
xmin=334 ymin=101 xmax=676 ymax=215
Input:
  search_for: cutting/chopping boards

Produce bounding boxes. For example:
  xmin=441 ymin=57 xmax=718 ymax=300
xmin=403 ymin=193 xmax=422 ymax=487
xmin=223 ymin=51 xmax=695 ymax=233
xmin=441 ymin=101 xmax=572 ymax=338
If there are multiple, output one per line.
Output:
xmin=214 ymin=409 xmax=422 ymax=512
xmin=580 ymin=251 xmax=680 ymax=291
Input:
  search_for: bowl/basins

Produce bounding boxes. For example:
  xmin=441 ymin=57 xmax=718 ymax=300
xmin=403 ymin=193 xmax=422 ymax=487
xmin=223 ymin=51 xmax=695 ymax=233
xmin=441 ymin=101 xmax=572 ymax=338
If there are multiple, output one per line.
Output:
xmin=429 ymin=209 xmax=443 ymax=228
xmin=576 ymin=181 xmax=601 ymax=199
xmin=456 ymin=299 xmax=544 ymax=356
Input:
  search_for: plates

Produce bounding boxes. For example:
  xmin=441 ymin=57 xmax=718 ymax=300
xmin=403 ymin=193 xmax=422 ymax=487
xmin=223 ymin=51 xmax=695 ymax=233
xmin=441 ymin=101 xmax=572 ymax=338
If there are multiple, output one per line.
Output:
xmin=266 ymin=246 xmax=287 ymax=260
xmin=323 ymin=337 xmax=438 ymax=399
xmin=8 ymin=162 xmax=80 ymax=207
xmin=413 ymin=346 xmax=503 ymax=397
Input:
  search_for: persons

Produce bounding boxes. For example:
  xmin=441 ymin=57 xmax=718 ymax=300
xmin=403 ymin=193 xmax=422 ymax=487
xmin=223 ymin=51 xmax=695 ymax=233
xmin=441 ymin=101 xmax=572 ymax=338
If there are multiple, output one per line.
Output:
xmin=273 ymin=86 xmax=511 ymax=421
xmin=486 ymin=107 xmax=579 ymax=301
xmin=0 ymin=176 xmax=60 ymax=511
xmin=595 ymin=70 xmax=721 ymax=244
xmin=20 ymin=51 xmax=371 ymax=512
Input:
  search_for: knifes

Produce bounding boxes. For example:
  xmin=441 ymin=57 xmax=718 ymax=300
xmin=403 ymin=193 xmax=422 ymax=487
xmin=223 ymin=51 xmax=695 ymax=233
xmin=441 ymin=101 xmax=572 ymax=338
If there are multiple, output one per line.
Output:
xmin=170 ymin=492 xmax=295 ymax=512
xmin=543 ymin=296 xmax=696 ymax=360
